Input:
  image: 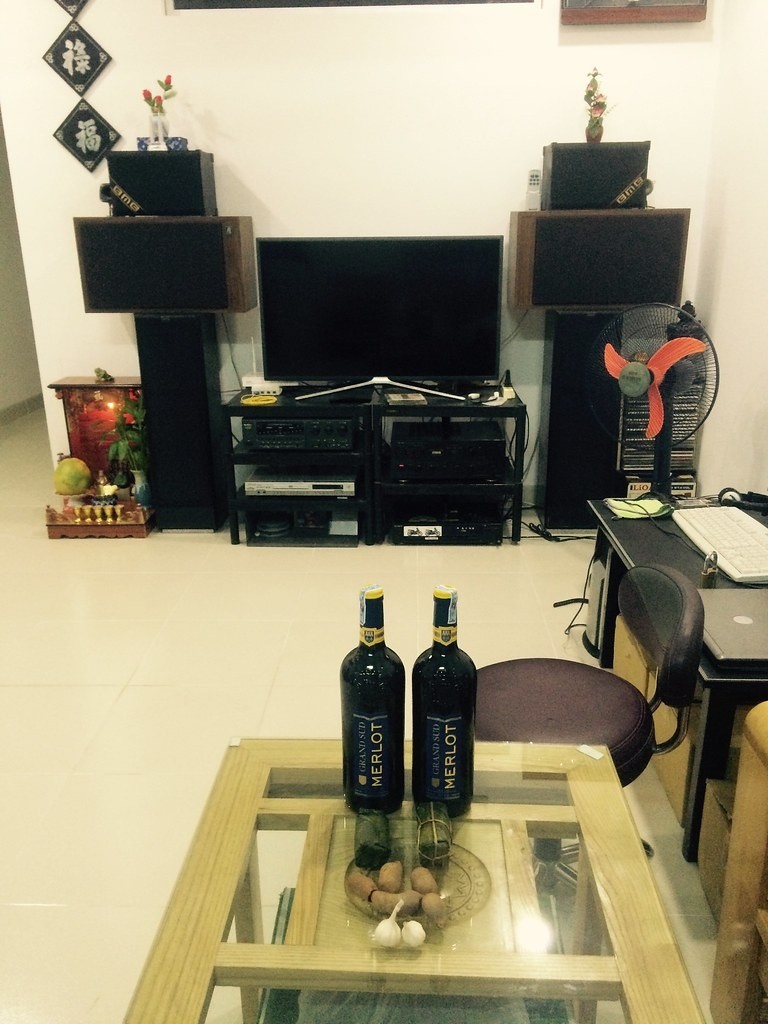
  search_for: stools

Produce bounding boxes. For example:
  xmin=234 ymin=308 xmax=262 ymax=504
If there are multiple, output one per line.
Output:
xmin=469 ymin=564 xmax=705 ymax=785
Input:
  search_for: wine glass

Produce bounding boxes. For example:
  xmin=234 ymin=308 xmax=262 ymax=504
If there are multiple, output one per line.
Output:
xmin=72 ymin=504 xmax=125 ymax=522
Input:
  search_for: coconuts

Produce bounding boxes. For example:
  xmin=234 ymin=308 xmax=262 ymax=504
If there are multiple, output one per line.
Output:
xmin=53 ymin=458 xmax=91 ymax=496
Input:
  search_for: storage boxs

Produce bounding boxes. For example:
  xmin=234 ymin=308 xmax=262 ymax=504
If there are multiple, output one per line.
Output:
xmin=697 ymin=778 xmax=741 ymax=923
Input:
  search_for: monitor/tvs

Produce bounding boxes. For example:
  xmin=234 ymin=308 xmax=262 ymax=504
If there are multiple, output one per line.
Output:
xmin=255 ymin=237 xmax=503 ymax=400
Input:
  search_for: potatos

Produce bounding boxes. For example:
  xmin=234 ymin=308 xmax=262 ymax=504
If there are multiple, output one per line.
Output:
xmin=345 ymin=859 xmax=448 ymax=929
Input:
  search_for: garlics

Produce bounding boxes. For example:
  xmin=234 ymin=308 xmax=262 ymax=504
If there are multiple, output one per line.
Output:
xmin=374 ymin=899 xmax=426 ymax=947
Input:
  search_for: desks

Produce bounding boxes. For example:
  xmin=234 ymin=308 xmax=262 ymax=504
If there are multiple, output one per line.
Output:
xmin=125 ymin=736 xmax=707 ymax=1023
xmin=587 ymin=494 xmax=768 ymax=860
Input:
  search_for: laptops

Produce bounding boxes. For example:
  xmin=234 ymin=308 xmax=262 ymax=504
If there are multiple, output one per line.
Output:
xmin=696 ymin=588 xmax=768 ymax=672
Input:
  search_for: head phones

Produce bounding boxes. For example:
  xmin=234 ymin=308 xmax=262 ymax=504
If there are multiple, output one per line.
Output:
xmin=718 ymin=488 xmax=768 ymax=516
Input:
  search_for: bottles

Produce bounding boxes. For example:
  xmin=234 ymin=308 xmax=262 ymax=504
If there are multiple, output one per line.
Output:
xmin=413 ymin=584 xmax=477 ymax=818
xmin=339 ymin=583 xmax=405 ymax=815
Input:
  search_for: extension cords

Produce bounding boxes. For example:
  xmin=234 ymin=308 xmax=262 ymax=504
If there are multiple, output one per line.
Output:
xmin=501 ymin=384 xmax=515 ymax=399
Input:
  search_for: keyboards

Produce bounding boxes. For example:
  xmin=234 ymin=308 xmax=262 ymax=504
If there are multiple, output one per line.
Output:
xmin=671 ymin=506 xmax=768 ymax=583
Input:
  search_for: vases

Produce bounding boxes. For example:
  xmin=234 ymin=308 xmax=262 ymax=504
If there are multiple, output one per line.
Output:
xmin=130 ymin=472 xmax=150 ymax=506
xmin=586 ymin=124 xmax=603 ymax=142
xmin=150 ymin=113 xmax=168 ymax=136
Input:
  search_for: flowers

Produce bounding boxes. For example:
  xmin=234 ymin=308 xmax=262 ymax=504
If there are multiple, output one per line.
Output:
xmin=586 ymin=68 xmax=606 ymax=123
xmin=108 ymin=391 xmax=145 ymax=467
xmin=142 ymin=75 xmax=172 ymax=112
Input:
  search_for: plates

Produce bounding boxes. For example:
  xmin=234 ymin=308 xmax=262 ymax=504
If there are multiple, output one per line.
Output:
xmin=53 ymin=488 xmax=91 ymax=508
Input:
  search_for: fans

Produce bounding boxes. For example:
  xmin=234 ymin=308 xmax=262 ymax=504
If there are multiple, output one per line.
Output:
xmin=588 ymin=303 xmax=720 ymax=493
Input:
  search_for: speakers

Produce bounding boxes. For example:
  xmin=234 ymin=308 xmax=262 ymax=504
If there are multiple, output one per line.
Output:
xmin=507 ymin=141 xmax=692 ymax=528
xmin=73 ymin=149 xmax=258 ymax=532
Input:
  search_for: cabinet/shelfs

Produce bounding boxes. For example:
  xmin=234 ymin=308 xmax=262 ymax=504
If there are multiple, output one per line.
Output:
xmin=220 ymin=383 xmax=527 ymax=544
